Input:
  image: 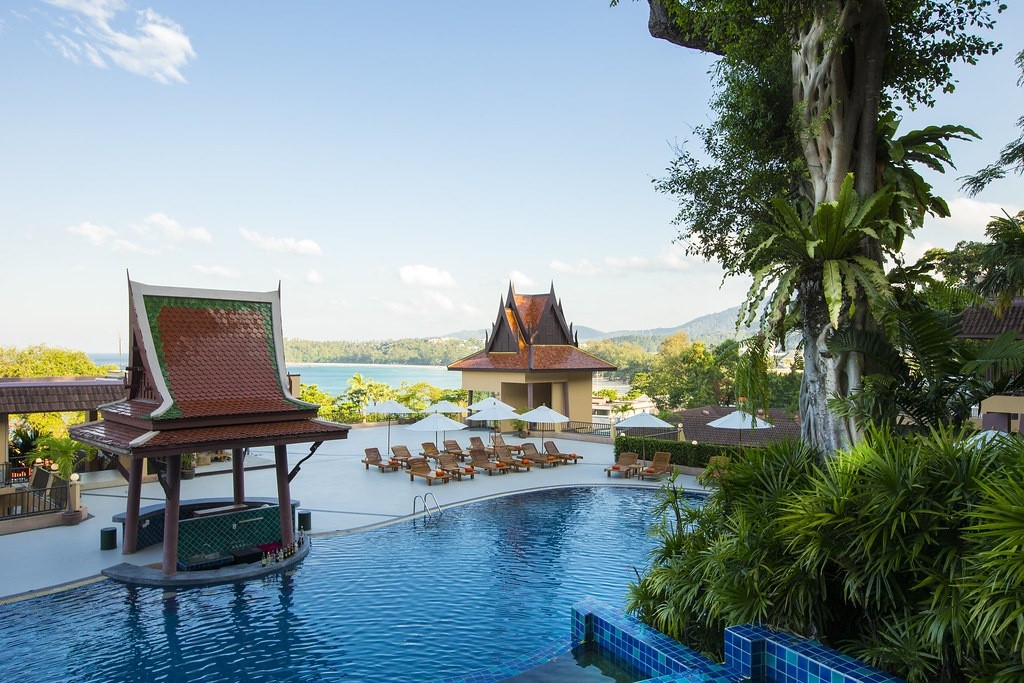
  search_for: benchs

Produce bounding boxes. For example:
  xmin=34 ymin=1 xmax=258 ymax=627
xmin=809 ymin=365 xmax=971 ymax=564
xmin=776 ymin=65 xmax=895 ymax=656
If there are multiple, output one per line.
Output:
xmin=176 ymin=546 xmax=263 ymax=571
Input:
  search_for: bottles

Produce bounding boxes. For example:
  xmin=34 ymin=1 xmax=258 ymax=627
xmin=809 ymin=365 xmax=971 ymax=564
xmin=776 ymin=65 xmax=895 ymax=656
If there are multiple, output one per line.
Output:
xmin=284 ymin=540 xmax=298 ymax=559
xmin=266 ymin=552 xmax=271 ymax=565
xmin=279 ymin=549 xmax=284 ymax=562
xmin=275 ymin=549 xmax=279 ymax=562
xmin=262 ymin=552 xmax=266 ymax=567
xmin=271 ymin=550 xmax=275 ymax=564
xmin=299 ymin=526 xmax=304 ymax=546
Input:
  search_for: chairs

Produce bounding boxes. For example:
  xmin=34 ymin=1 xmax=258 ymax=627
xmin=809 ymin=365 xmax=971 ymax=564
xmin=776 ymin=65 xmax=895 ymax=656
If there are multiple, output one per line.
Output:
xmin=361 ymin=434 xmax=584 ymax=486
xmin=637 ymin=452 xmax=673 ymax=482
xmin=697 ymin=455 xmax=731 ymax=485
xmin=605 ymin=453 xmax=640 ymax=479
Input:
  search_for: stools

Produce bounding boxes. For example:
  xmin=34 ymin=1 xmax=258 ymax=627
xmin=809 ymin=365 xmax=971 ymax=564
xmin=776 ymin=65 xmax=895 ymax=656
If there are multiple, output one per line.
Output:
xmin=298 ymin=510 xmax=312 ymax=532
xmin=101 ymin=526 xmax=117 ymax=550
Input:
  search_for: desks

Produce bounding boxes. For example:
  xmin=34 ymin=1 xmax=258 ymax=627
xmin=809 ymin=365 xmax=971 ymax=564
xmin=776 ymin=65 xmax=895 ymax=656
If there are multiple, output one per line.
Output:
xmin=629 ymin=464 xmax=645 ymax=480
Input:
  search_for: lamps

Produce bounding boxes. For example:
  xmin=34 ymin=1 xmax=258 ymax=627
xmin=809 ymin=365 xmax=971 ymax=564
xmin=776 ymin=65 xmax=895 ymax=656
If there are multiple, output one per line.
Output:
xmin=678 ymin=423 xmax=684 ymax=428
xmin=69 ymin=473 xmax=79 ymax=481
xmin=34 ymin=457 xmax=42 ymax=465
xmin=51 ymin=463 xmax=60 ymax=471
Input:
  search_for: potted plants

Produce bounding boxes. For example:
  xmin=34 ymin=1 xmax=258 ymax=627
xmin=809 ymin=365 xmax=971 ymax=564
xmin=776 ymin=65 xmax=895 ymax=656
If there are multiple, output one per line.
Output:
xmin=182 ymin=452 xmax=196 ymax=479
xmin=23 ymin=435 xmax=101 ymax=526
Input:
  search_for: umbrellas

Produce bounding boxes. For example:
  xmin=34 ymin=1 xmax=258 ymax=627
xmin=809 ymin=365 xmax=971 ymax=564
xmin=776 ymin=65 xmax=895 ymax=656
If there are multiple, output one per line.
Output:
xmin=517 ymin=403 xmax=571 ymax=453
xmin=407 ymin=400 xmax=469 ymax=454
xmin=364 ymin=398 xmax=417 ymax=454
xmin=467 ymin=397 xmax=516 ymax=444
xmin=967 ymin=425 xmax=1015 ymax=451
xmin=466 ymin=402 xmax=520 ymax=456
xmin=614 ymin=409 xmax=675 ymax=466
xmin=706 ymin=410 xmax=775 ymax=462
